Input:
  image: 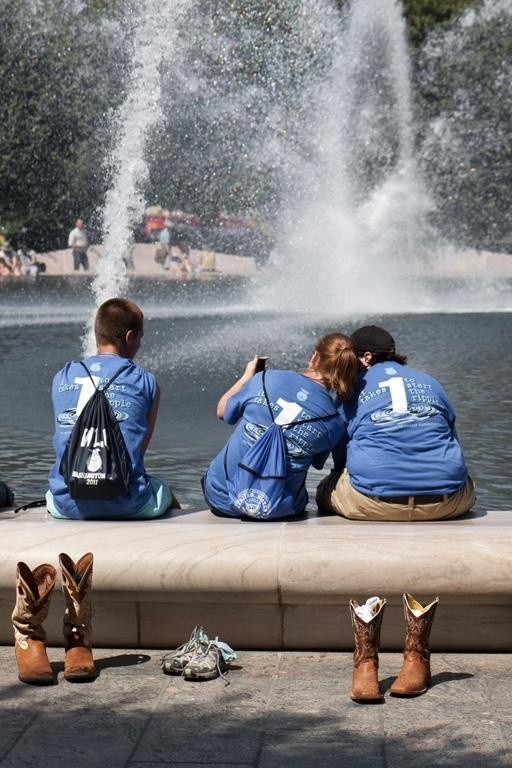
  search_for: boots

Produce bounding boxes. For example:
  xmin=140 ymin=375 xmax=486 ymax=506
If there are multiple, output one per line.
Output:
xmin=390 ymin=593 xmax=438 ymax=695
xmin=349 ymin=598 xmax=387 ymax=702
xmin=11 ymin=562 xmax=57 ymax=684
xmin=59 ymin=553 xmax=96 ymax=682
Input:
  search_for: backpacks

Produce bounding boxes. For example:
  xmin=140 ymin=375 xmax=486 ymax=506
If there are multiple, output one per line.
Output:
xmin=58 ymin=362 xmax=132 ymax=502
xmin=224 ymin=370 xmax=341 ymax=519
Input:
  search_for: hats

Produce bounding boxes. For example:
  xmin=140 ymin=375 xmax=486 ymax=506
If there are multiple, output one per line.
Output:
xmin=353 ymin=325 xmax=395 ymax=353
xmin=0 ymin=243 xmax=12 ymax=250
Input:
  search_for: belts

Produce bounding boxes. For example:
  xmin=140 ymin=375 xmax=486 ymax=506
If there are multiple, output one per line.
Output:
xmin=371 ymin=490 xmax=457 ymax=504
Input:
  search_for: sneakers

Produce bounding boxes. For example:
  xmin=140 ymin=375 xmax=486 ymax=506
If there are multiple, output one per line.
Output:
xmin=182 ymin=636 xmax=230 ymax=680
xmin=159 ymin=624 xmax=208 ymax=673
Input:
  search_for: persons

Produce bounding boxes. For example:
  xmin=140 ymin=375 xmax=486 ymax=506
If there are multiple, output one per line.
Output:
xmin=45 ymin=298 xmax=181 ymax=519
xmin=67 ymin=217 xmax=90 ymax=272
xmin=16 ymin=243 xmax=39 ymax=278
xmin=201 ymin=332 xmax=358 ymax=519
xmin=0 ymin=240 xmax=20 ymax=277
xmin=122 ymin=218 xmax=216 ymax=276
xmin=316 ymin=325 xmax=477 ymax=521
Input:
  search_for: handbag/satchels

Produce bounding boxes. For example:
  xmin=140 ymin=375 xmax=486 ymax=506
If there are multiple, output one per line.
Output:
xmin=154 ymin=246 xmax=169 ymax=263
xmin=22 ymin=250 xmax=46 ymax=272
